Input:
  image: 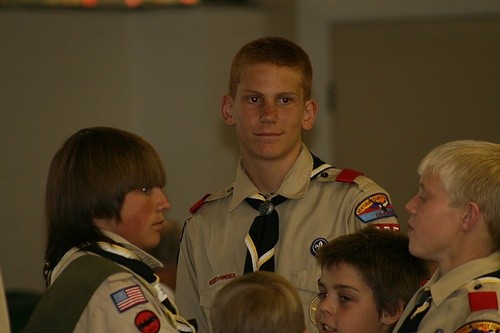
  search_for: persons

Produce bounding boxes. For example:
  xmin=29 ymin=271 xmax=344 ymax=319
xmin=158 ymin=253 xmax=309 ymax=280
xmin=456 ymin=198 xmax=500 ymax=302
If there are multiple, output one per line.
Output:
xmin=314 ymin=225 xmax=430 ymax=333
xmin=391 ymin=139 xmax=500 ymax=333
xmin=208 ymin=271 xmax=308 ymax=333
xmin=25 ymin=126 xmax=197 ymax=333
xmin=175 ymin=36 xmax=399 ymax=333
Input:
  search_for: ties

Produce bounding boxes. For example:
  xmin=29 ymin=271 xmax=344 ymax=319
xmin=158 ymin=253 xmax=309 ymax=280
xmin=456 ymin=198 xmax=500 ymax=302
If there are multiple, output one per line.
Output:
xmin=397 ymin=270 xmax=500 ymax=333
xmin=242 ymin=151 xmax=335 ymax=273
xmin=43 ymin=229 xmax=196 ymax=333
